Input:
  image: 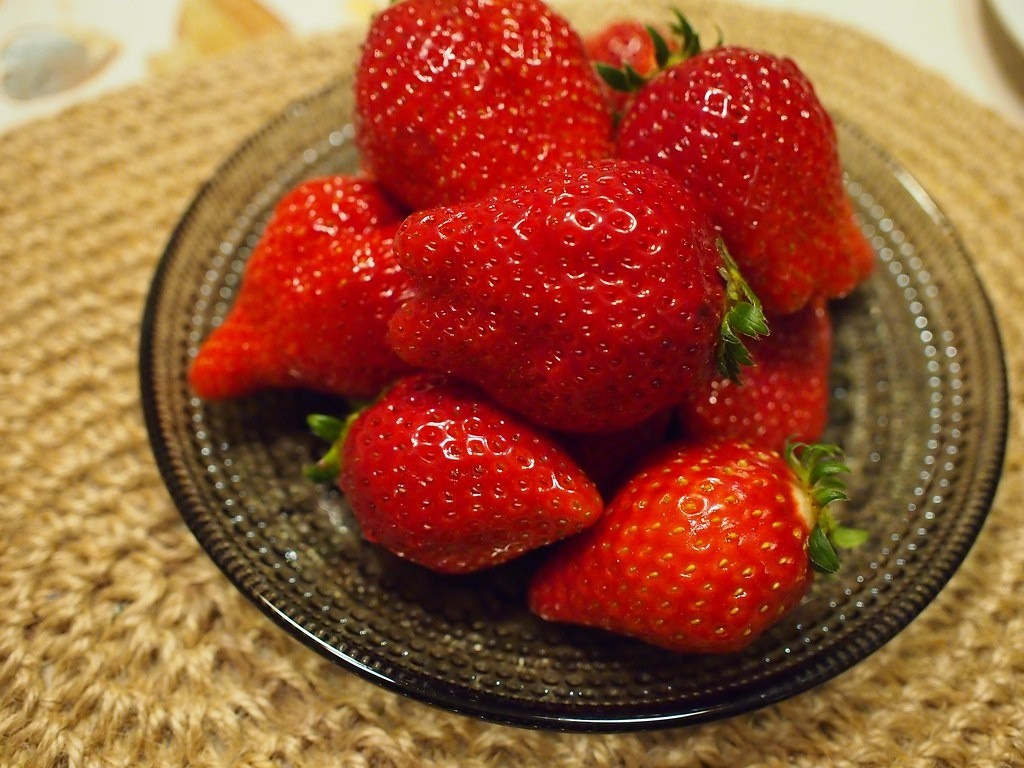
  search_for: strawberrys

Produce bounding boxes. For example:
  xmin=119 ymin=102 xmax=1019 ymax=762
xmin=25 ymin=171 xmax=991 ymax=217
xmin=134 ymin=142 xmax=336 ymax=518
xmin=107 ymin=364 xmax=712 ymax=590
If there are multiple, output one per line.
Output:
xmin=191 ymin=0 xmax=878 ymax=654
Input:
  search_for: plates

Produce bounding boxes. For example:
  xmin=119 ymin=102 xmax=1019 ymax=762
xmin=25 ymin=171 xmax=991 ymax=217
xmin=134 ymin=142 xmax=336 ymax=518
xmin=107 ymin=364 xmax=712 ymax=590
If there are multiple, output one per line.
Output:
xmin=136 ymin=66 xmax=1009 ymax=733
